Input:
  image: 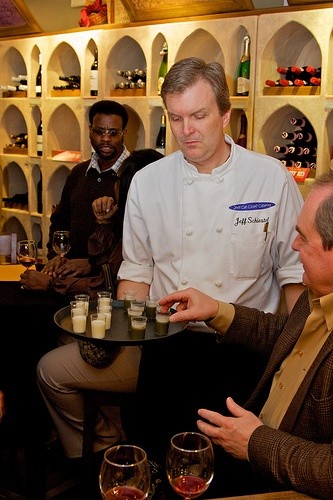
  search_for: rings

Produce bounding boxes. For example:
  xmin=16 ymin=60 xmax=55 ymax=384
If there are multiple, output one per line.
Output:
xmin=20 ymin=285 xmax=24 ymax=289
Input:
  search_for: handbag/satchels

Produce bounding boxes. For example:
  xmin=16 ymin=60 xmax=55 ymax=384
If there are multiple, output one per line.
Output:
xmin=76 ymin=264 xmax=121 ymax=368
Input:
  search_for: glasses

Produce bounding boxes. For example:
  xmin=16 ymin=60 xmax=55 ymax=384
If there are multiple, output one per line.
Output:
xmin=90 ymin=125 xmax=124 ymax=137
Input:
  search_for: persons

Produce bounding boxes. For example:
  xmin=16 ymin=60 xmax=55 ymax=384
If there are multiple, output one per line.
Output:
xmin=41 ymin=100 xmax=132 ymax=278
xmin=20 ymin=149 xmax=165 ymax=473
xmin=159 ymin=171 xmax=333 ymax=500
xmin=116 ymin=56 xmax=308 ymax=456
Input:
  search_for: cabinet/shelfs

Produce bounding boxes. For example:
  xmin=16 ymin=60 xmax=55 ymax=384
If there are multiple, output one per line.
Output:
xmin=0 ymin=0 xmax=333 ymax=262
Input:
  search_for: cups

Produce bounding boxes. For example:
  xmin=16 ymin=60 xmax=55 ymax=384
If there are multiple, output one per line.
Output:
xmin=124 ymin=290 xmax=171 ymax=340
xmin=98 ymin=445 xmax=151 ymax=500
xmin=70 ymin=291 xmax=113 ymax=339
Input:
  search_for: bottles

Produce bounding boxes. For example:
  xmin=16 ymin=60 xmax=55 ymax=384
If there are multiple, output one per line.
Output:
xmin=158 ymin=41 xmax=168 ymax=96
xmin=2 ymin=171 xmax=59 ymax=214
xmin=112 ymin=68 xmax=146 ymax=90
xmin=265 ymin=65 xmax=321 ymax=87
xmin=235 ymin=113 xmax=247 ymax=148
xmin=6 ymin=133 xmax=28 ymax=149
xmin=155 ymin=115 xmax=166 ymax=156
xmin=0 ymin=74 xmax=27 ymax=92
xmin=236 ymin=35 xmax=250 ymax=96
xmin=90 ymin=48 xmax=98 ymax=97
xmin=35 ymin=54 xmax=42 ymax=97
xmin=273 ymin=116 xmax=317 ymax=171
xmin=53 ymin=74 xmax=80 ymax=90
xmin=37 ymin=116 xmax=43 ymax=156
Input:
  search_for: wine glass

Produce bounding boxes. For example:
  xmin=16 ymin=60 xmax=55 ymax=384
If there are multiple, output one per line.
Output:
xmin=16 ymin=240 xmax=37 ymax=289
xmin=52 ymin=231 xmax=72 ymax=268
xmin=166 ymin=432 xmax=215 ymax=500
